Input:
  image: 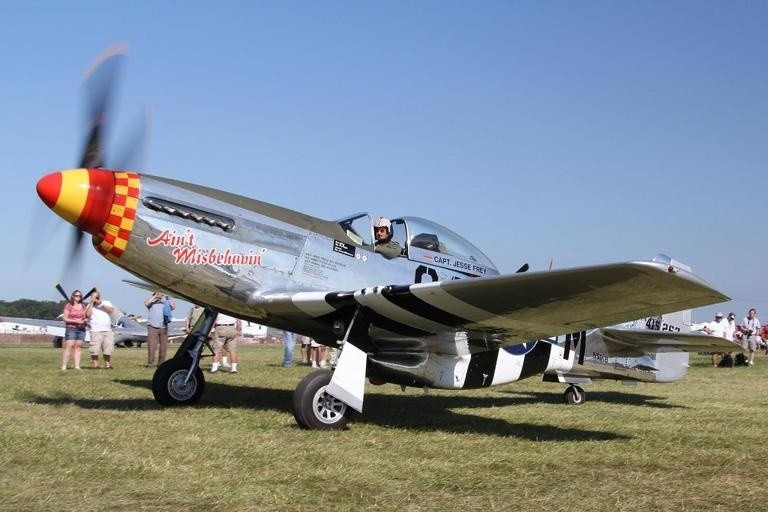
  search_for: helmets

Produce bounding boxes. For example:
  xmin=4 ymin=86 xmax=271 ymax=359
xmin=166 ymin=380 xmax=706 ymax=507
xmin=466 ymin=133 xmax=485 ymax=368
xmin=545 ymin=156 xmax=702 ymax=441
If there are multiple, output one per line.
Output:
xmin=374 ymin=218 xmax=393 ymax=240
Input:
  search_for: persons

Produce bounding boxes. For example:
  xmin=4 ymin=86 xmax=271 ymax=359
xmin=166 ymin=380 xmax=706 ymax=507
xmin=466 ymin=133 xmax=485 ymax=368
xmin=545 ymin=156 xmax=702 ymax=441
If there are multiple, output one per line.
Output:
xmin=720 ymin=313 xmax=739 ymax=357
xmin=61 ymin=290 xmax=88 ymax=370
xmin=362 ymin=217 xmax=402 ymax=258
xmin=740 ymin=309 xmax=761 ymax=365
xmin=186 ymin=304 xmax=241 ymax=374
xmin=84 ymin=292 xmax=114 ymax=369
xmin=761 ymin=324 xmax=768 ymax=357
xmin=144 ymin=293 xmax=176 ymax=368
xmin=281 ymin=329 xmax=340 ymax=369
xmin=704 ymin=312 xmax=724 ymax=367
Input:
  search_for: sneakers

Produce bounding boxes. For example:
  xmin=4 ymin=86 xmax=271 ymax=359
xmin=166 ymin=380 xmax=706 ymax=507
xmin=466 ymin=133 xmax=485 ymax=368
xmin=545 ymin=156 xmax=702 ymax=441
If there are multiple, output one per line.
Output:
xmin=61 ymin=362 xmax=112 ymax=369
xmin=302 ymin=358 xmax=328 ymax=368
xmin=147 ymin=361 xmax=163 ymax=367
xmin=211 ymin=363 xmax=238 ymax=373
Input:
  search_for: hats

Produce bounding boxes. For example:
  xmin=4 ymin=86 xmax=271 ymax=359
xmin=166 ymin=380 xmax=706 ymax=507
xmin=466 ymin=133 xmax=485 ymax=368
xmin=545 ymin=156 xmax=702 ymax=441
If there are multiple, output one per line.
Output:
xmin=715 ymin=312 xmax=722 ymax=317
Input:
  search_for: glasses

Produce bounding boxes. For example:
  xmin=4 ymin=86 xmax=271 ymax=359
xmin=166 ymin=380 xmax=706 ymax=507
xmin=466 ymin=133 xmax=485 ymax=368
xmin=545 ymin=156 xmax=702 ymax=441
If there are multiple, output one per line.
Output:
xmin=74 ymin=295 xmax=81 ymax=297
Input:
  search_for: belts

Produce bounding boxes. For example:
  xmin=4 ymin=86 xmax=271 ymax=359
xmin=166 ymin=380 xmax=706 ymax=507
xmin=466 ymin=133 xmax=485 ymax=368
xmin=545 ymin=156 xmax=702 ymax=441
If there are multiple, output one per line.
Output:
xmin=215 ymin=324 xmax=235 ymax=326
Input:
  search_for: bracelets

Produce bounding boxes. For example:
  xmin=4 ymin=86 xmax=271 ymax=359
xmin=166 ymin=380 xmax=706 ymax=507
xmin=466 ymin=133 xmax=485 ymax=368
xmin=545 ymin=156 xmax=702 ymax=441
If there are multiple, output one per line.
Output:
xmin=99 ymin=303 xmax=104 ymax=307
